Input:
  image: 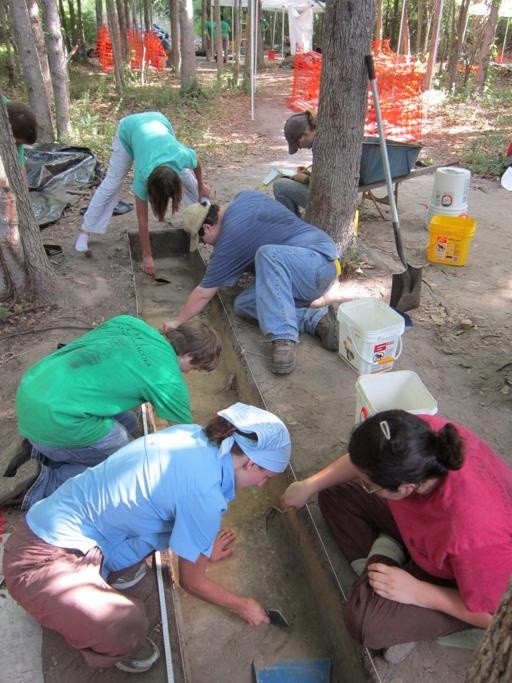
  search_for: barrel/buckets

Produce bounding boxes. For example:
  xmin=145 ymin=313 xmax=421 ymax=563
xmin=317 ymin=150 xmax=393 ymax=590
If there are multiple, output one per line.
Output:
xmin=338 ymin=296 xmax=405 ymax=372
xmin=355 ymin=371 xmax=440 ymax=421
xmin=428 ymin=213 xmax=477 ymax=267
xmin=425 ymin=167 xmax=470 ymax=236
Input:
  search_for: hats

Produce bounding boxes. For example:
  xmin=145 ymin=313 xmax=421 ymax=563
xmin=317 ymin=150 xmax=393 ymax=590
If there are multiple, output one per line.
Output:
xmin=181 ymin=200 xmax=211 ymax=252
xmin=284 ymin=112 xmax=308 ymax=154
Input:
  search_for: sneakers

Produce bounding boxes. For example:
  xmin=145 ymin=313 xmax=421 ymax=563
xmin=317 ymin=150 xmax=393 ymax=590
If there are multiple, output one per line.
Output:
xmin=315 ymin=305 xmax=339 ymax=351
xmin=114 ymin=636 xmax=161 ymax=673
xmin=270 ymin=340 xmax=298 ymax=374
xmin=106 ymin=558 xmax=148 ymax=590
xmin=0 ymin=433 xmax=42 ymax=505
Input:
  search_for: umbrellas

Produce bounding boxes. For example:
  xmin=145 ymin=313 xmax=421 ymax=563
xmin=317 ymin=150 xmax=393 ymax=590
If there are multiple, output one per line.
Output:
xmin=261 ymin=0 xmax=326 ymax=59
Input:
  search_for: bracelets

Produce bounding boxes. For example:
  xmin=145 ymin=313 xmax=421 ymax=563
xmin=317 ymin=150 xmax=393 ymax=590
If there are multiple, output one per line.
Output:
xmin=303 ymin=174 xmax=308 ymax=185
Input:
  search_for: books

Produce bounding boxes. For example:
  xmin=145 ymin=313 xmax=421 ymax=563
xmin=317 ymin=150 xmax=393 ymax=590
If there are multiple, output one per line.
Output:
xmin=262 ymin=167 xmax=298 ymax=185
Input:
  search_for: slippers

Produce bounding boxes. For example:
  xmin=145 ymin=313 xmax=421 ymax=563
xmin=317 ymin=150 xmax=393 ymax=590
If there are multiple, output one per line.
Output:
xmin=113 ymin=200 xmax=133 ymax=215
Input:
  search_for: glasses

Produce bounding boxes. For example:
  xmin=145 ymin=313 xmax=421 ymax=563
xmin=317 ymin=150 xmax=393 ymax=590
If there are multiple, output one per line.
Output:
xmin=358 ymin=479 xmax=384 ymax=494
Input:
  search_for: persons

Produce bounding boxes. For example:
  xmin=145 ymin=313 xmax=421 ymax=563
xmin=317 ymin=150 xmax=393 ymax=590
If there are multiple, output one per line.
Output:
xmin=261 ymin=15 xmax=270 ymax=44
xmin=203 ymin=13 xmax=216 ymax=63
xmin=280 ymin=405 xmax=511 ymax=663
xmin=2 ymin=99 xmax=41 ymax=244
xmin=213 ymin=14 xmax=232 ymax=62
xmin=0 ymin=311 xmax=221 ymax=510
xmin=158 ymin=186 xmax=340 ymax=371
xmin=73 ymin=110 xmax=213 ymax=278
xmin=1 ymin=401 xmax=290 ymax=674
xmin=275 ymin=112 xmax=319 ymax=214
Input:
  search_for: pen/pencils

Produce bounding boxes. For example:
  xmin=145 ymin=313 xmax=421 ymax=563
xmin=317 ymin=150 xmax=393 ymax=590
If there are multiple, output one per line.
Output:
xmin=305 ymin=165 xmax=311 ymax=170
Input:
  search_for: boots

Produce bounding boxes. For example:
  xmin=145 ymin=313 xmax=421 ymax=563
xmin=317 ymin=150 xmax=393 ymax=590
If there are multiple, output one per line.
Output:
xmin=350 ymin=532 xmax=408 ymax=577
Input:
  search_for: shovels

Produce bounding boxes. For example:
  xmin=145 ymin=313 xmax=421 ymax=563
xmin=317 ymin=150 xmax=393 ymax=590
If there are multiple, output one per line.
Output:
xmin=264 ymin=607 xmax=289 ymax=627
xmin=366 ymin=54 xmax=422 ymax=312
xmin=266 ymin=506 xmax=286 ymax=528
xmin=150 ymin=273 xmax=172 ymax=284
